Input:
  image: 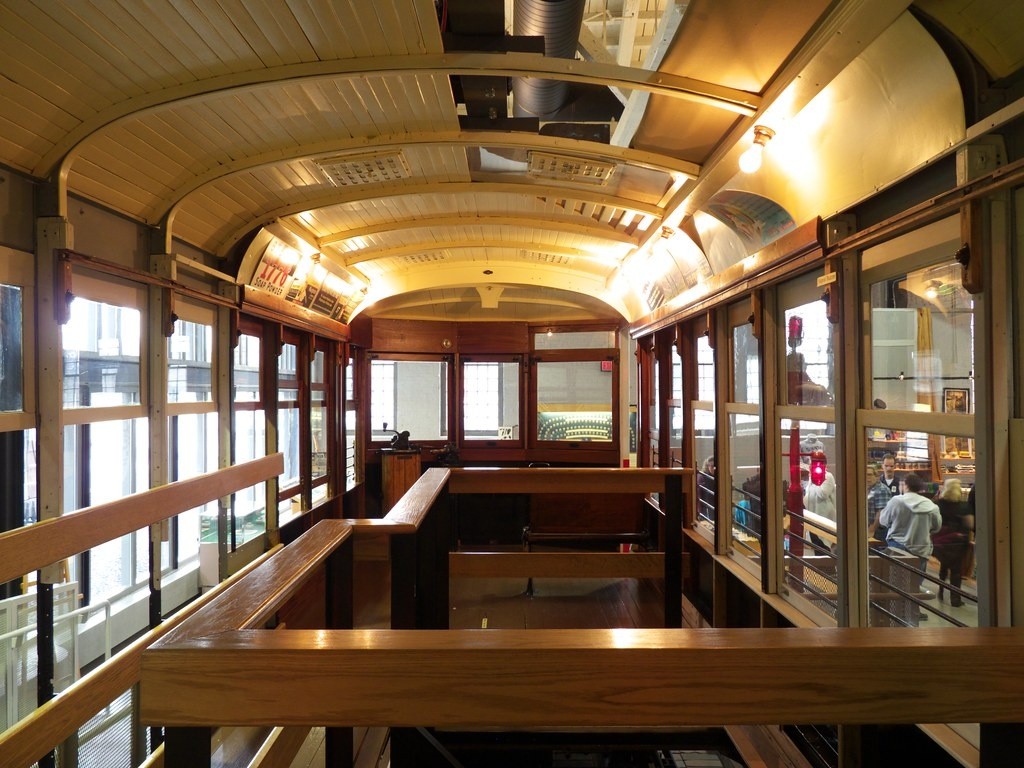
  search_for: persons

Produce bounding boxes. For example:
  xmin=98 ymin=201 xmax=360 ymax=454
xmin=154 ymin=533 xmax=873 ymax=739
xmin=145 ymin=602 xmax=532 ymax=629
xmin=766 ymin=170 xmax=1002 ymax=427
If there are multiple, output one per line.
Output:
xmin=800 ymin=458 xmax=836 ymax=556
xmin=742 ymin=468 xmax=759 ymax=534
xmin=931 ymin=479 xmax=975 ymax=608
xmin=880 ymin=473 xmax=942 ymax=620
xmin=698 ymin=456 xmax=714 ymax=519
xmin=866 ymin=467 xmax=892 ymax=541
xmin=879 ymin=453 xmax=901 ymax=495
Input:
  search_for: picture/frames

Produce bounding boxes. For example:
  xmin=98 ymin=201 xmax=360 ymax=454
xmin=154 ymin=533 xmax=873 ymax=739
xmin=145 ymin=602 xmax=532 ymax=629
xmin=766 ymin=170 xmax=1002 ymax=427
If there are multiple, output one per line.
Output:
xmin=942 ymin=387 xmax=970 ymax=414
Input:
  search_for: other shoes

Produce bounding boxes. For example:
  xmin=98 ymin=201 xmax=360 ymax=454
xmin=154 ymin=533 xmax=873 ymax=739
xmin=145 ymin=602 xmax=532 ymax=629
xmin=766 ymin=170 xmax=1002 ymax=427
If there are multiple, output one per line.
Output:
xmin=919 ymin=613 xmax=928 ymax=620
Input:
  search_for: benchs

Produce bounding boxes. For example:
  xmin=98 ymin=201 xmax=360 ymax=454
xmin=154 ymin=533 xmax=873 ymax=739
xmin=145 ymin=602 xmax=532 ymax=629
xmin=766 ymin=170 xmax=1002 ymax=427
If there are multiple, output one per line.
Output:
xmin=523 ymin=462 xmax=652 ymax=596
